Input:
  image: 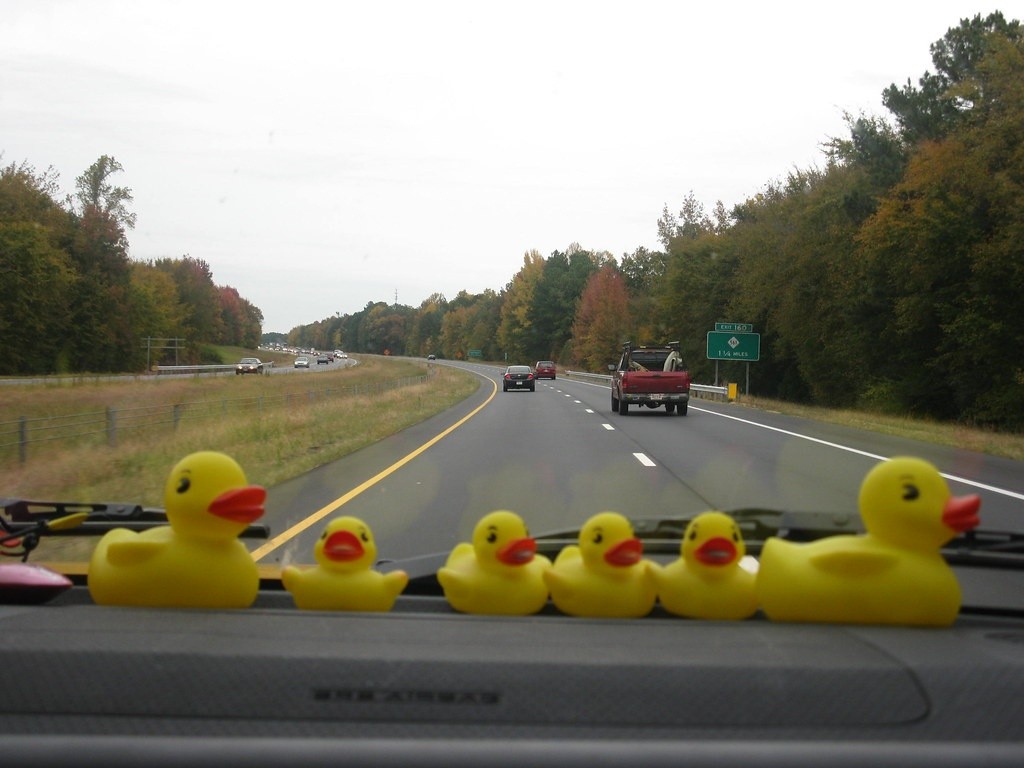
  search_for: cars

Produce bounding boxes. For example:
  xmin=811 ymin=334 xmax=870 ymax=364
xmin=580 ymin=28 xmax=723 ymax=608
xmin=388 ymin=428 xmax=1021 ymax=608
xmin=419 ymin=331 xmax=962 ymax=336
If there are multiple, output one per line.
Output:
xmin=324 ymin=353 xmax=335 ymax=362
xmin=316 ymin=354 xmax=329 ymax=363
xmin=259 ymin=342 xmax=348 ymax=359
xmin=236 ymin=357 xmax=263 ymax=375
xmin=534 ymin=361 xmax=557 ymax=379
xmin=500 ymin=365 xmax=535 ymax=391
xmin=294 ymin=356 xmax=310 ymax=368
xmin=427 ymin=354 xmax=435 ymax=360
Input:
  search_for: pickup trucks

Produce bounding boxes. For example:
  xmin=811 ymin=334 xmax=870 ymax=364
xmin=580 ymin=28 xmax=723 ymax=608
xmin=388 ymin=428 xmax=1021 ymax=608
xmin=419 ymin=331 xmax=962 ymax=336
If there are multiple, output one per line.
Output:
xmin=606 ymin=340 xmax=692 ymax=416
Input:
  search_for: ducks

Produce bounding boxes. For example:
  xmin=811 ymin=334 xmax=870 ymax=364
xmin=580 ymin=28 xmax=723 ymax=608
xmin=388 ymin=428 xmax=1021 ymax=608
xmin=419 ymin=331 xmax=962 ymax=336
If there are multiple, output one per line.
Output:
xmin=89 ymin=449 xmax=265 ymax=607
xmin=436 ymin=456 xmax=982 ymax=627
xmin=281 ymin=515 xmax=410 ymax=613
